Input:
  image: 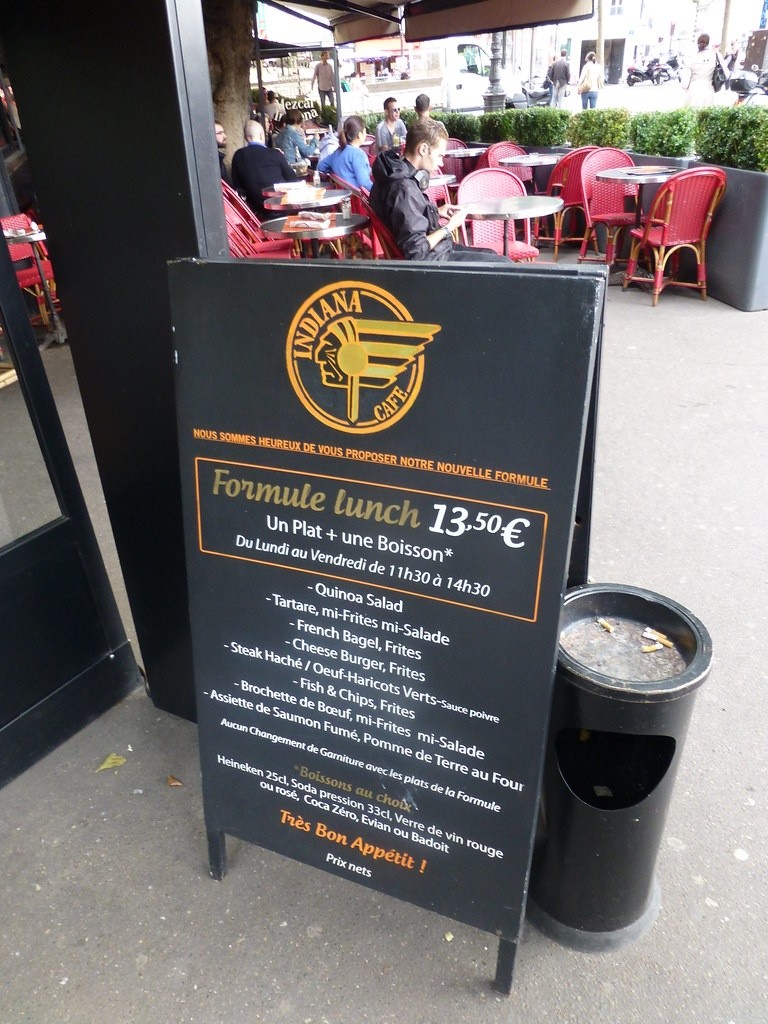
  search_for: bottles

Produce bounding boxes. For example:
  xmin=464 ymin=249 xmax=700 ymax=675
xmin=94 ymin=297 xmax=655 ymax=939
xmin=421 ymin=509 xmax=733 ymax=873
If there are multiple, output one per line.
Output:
xmin=341 ymin=197 xmax=351 ymax=219
xmin=312 ymin=171 xmax=320 ymax=185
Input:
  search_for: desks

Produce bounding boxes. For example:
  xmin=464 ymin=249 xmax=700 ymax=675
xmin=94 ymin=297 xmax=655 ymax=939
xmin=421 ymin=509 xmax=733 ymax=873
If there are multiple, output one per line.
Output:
xmin=443 ymin=149 xmax=486 ymax=163
xmin=6 ymin=231 xmax=68 ymax=351
xmin=427 ymin=174 xmax=457 ymax=193
xmin=258 ymin=212 xmax=372 ymax=257
xmin=498 ymin=155 xmax=570 ymax=189
xmin=264 ymin=188 xmax=353 ymax=212
xmin=450 ymin=195 xmax=565 ymax=256
xmin=260 ymin=182 xmax=334 ymax=194
xmin=594 ymin=167 xmax=686 ymax=230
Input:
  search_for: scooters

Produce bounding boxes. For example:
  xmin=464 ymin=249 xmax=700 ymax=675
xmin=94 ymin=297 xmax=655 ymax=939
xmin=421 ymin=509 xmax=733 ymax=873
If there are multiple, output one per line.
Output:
xmin=518 ymin=65 xmax=571 ymax=108
xmin=729 ymin=63 xmax=768 ymax=110
xmin=626 ymin=49 xmax=685 ymax=87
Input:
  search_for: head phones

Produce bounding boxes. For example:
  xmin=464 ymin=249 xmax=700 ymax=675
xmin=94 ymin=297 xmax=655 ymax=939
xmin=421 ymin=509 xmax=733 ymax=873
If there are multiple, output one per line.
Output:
xmin=403 ymin=157 xmax=429 ymax=191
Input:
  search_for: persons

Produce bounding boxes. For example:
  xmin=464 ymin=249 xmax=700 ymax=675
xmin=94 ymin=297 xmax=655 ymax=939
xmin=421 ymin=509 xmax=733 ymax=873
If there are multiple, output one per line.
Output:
xmin=215 ymin=120 xmax=228 ymax=180
xmin=549 ymin=49 xmax=570 ymax=109
xmin=414 ymin=94 xmax=432 ymax=119
xmin=231 ymin=119 xmax=298 ymax=223
xmin=311 ymin=50 xmax=335 ymax=108
xmin=681 ymin=34 xmax=730 ymax=107
xmin=382 ymin=67 xmax=389 ymax=77
xmin=375 ymin=97 xmax=408 ymax=153
xmin=577 ymin=51 xmax=602 ymax=109
xmin=724 ymin=38 xmax=738 ymax=89
xmin=315 ymin=116 xmax=374 ymax=190
xmin=367 ymin=119 xmax=512 ymax=263
xmin=350 ymin=72 xmax=370 ymax=111
xmin=256 ymin=87 xmax=320 ymax=163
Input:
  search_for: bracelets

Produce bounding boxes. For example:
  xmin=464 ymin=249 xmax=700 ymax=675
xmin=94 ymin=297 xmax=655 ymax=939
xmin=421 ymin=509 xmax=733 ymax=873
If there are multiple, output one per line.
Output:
xmin=441 ymin=225 xmax=449 ymax=234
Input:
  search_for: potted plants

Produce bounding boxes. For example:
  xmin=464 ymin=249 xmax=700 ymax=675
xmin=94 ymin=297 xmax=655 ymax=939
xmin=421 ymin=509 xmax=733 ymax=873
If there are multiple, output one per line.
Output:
xmin=356 ymin=105 xmax=768 ymax=312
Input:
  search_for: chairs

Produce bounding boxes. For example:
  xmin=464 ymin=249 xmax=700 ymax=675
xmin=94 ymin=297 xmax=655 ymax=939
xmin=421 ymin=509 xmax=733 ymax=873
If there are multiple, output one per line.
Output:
xmin=1 ymin=214 xmax=62 ymax=323
xmin=222 ymin=103 xmax=728 ymax=308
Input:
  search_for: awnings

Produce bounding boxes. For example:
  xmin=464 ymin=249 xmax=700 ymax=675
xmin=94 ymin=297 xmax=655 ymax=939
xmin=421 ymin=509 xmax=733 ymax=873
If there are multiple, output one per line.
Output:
xmin=253 ymin=0 xmax=402 ymax=146
xmin=324 ymin=0 xmax=594 ymax=42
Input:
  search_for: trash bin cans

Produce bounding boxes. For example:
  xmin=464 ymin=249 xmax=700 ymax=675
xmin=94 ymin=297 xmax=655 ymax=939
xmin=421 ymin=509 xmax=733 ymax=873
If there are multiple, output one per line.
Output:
xmin=526 ymin=583 xmax=713 ymax=954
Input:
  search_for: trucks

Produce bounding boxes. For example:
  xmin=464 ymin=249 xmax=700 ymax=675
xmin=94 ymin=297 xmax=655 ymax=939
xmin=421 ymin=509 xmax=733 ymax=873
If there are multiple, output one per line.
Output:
xmin=334 ymin=33 xmax=528 ymax=119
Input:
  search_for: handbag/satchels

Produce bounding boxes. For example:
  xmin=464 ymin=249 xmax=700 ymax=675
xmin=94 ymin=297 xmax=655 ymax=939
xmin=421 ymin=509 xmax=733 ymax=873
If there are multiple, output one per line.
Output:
xmin=578 ymin=63 xmax=591 ymax=95
xmin=712 ymin=52 xmax=726 ymax=93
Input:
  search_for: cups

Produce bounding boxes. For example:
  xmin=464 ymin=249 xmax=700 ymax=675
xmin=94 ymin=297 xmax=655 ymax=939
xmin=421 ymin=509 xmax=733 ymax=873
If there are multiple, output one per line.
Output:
xmin=392 ymin=133 xmax=399 ymax=147
xmin=401 ymin=136 xmax=406 ymax=147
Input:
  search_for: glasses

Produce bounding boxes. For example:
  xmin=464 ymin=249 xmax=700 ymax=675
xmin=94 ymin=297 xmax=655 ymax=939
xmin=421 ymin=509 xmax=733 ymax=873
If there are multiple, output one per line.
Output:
xmin=217 ymin=131 xmax=226 ymax=135
xmin=389 ymin=108 xmax=401 ymax=113
